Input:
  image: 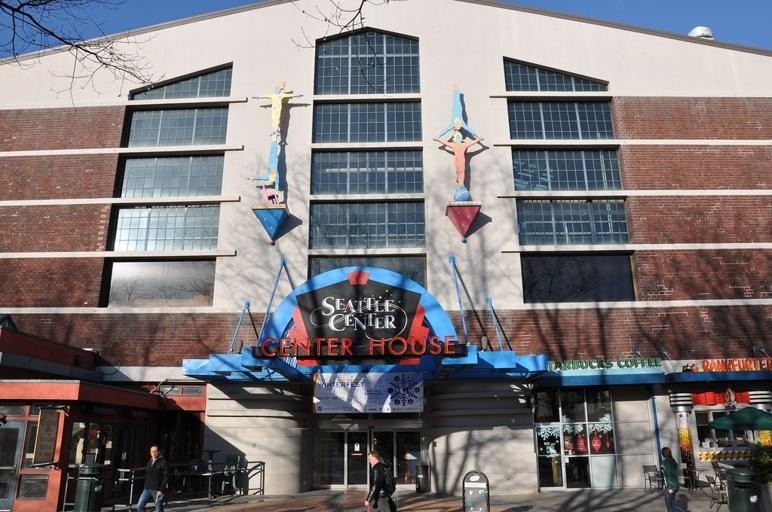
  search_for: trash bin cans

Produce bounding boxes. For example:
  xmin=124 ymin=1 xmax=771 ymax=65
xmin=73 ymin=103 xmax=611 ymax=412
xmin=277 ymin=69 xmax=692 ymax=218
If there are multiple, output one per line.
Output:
xmin=727 ymin=468 xmax=761 ymax=512
xmin=72 ymin=463 xmax=105 ymax=512
xmin=415 ymin=465 xmax=428 ymax=493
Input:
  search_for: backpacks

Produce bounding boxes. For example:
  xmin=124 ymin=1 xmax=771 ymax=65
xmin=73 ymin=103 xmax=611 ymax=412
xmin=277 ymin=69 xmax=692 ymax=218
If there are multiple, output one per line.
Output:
xmin=383 ymin=468 xmax=397 ymax=492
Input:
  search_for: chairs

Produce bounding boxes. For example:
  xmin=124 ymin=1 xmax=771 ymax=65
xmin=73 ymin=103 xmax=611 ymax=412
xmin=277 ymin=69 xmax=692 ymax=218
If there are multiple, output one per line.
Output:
xmin=641 ymin=460 xmax=730 ymax=511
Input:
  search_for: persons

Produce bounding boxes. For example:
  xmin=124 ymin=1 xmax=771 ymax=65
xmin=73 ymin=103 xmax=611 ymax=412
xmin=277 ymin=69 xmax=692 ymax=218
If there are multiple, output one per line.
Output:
xmin=365 ymin=451 xmax=397 ymax=512
xmin=137 ymin=443 xmax=170 ymax=512
xmin=662 ymin=447 xmax=690 ymax=512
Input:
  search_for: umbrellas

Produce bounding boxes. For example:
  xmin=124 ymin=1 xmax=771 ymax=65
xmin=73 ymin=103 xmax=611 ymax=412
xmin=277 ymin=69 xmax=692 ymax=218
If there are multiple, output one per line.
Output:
xmin=709 ymin=407 xmax=772 ymax=445
